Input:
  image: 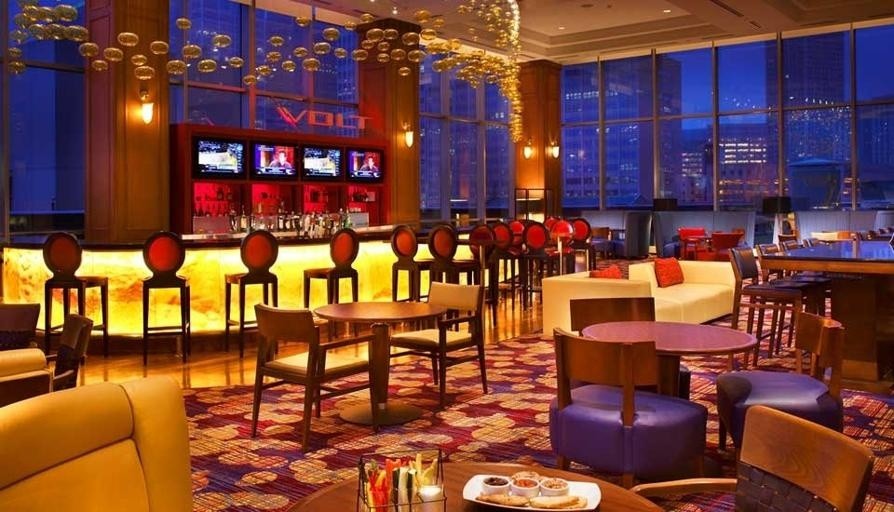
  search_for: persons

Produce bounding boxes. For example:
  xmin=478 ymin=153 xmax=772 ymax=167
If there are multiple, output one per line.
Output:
xmin=360 ymin=156 xmax=378 ymax=171
xmin=269 ymin=150 xmax=291 ymax=168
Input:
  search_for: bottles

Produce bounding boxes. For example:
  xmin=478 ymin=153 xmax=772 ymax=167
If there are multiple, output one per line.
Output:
xmin=196 ymin=187 xmax=368 ymax=232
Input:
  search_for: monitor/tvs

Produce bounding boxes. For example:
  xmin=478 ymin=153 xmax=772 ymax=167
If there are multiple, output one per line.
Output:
xmin=191 ymin=136 xmax=245 ymax=179
xmin=249 ymin=140 xmax=298 ymax=177
xmin=300 ymin=143 xmax=341 ymax=179
xmin=346 ymin=146 xmax=386 ymax=184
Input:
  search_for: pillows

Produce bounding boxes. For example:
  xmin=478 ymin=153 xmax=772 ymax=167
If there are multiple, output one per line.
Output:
xmin=653 ymin=257 xmax=685 ymax=288
xmin=589 ymin=263 xmax=623 ymax=278
xmin=602 ymin=260 xmax=630 ymax=280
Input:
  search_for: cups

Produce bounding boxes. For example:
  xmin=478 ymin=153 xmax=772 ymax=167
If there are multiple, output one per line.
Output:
xmin=483 ymin=476 xmax=510 ymax=493
xmin=421 ymin=483 xmax=443 ymax=512
xmin=509 ymin=479 xmax=539 ymax=497
xmin=540 ymin=476 xmax=568 ymax=498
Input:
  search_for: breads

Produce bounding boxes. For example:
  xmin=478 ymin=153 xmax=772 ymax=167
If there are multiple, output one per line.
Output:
xmin=474 ymin=492 xmax=587 ymax=510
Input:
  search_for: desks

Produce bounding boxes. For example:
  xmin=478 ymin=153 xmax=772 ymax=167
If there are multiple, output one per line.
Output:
xmin=313 ymin=300 xmax=449 ymax=411
xmin=579 ymin=320 xmax=761 ymax=398
xmin=760 ymin=239 xmax=894 ymax=383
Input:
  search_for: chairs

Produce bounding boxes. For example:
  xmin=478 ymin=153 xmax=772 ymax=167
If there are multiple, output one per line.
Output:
xmin=2 ymin=302 xmax=42 ymax=350
xmin=716 ymin=309 xmax=843 ymax=451
xmin=727 ymin=234 xmax=830 ymax=372
xmin=46 ymin=312 xmax=94 ymax=394
xmin=251 ymin=303 xmax=379 ymax=438
xmin=854 ymin=227 xmax=893 ymax=241
xmin=548 ymin=327 xmax=713 ymax=489
xmin=390 ymin=282 xmax=487 ymax=398
xmin=569 ymin=295 xmax=656 ymax=338
xmin=629 ymin=405 xmax=875 ymax=512
xmin=679 ymin=228 xmax=745 ymax=262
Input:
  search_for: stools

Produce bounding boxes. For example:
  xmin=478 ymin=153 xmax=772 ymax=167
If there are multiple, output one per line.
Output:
xmin=140 ymin=229 xmax=193 ymax=367
xmin=511 ymin=216 xmax=597 ymax=309
xmin=303 ymin=226 xmax=360 ymax=308
xmin=41 ymin=230 xmax=110 ymax=358
xmin=391 ymin=220 xmax=512 ymax=328
xmin=223 ymin=229 xmax=280 ymax=360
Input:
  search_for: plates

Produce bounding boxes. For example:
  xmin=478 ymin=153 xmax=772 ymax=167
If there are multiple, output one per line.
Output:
xmin=460 ymin=473 xmax=601 ymax=511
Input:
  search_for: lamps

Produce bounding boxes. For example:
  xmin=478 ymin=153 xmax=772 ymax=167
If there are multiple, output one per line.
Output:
xmin=551 ymin=140 xmax=560 ymax=159
xmin=523 ymin=137 xmax=532 ymax=159
xmin=138 ymin=88 xmax=154 ymax=124
xmin=402 ymin=119 xmax=414 ymax=148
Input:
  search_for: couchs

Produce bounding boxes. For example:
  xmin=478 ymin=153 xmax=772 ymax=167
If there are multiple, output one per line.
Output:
xmin=1 ymin=373 xmax=196 ymax=511
xmin=541 ymin=257 xmax=738 ymax=341
xmin=0 ymin=348 xmax=58 ymax=411
